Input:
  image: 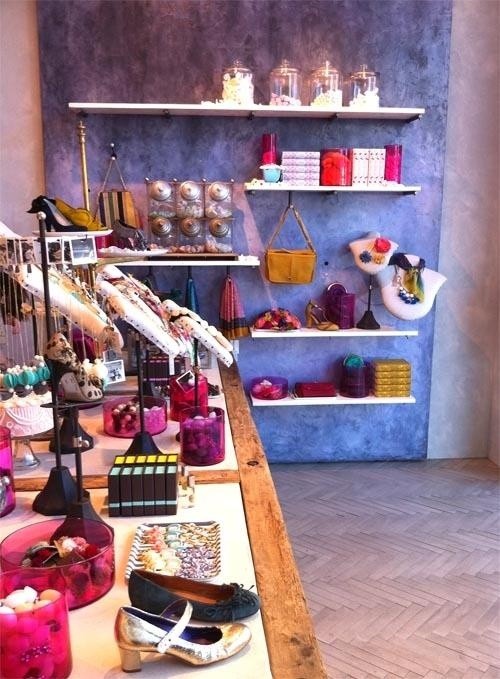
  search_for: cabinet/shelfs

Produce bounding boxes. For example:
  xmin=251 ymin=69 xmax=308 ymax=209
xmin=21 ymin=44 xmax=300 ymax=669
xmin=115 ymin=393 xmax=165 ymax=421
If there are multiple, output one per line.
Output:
xmin=68 ymin=102 xmax=426 ymax=406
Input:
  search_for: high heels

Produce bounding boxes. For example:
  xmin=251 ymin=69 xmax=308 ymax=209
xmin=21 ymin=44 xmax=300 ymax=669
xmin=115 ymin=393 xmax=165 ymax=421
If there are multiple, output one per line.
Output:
xmin=31 ymin=195 xmax=108 ymax=232
xmin=306 ymin=299 xmax=339 ymax=331
xmin=114 ymin=569 xmax=260 ymax=673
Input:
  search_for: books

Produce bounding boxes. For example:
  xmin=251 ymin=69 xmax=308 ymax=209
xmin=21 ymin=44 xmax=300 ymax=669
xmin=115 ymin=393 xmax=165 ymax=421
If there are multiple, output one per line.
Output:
xmin=351 ymin=147 xmax=387 ymax=186
xmin=371 ymin=358 xmax=412 ymax=397
xmin=278 ymin=147 xmax=321 ymax=185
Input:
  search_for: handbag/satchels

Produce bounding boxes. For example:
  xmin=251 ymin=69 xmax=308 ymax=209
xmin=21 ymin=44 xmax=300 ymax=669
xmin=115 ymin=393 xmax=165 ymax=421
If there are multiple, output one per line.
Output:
xmin=97 ymin=157 xmax=139 ymax=229
xmin=264 ymin=203 xmax=317 ymax=285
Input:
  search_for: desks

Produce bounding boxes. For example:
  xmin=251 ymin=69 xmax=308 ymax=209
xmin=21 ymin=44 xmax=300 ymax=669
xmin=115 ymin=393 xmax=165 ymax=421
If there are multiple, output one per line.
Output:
xmin=0 ymin=352 xmax=331 ymax=679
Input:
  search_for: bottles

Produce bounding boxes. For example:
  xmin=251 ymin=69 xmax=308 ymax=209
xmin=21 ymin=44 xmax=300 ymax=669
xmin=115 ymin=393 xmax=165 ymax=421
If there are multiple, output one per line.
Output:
xmin=347 ymin=63 xmax=381 ymax=108
xmin=307 ymin=60 xmax=343 ymax=107
xmin=266 ymin=59 xmax=302 ymax=107
xmin=219 ymin=60 xmax=256 ymax=104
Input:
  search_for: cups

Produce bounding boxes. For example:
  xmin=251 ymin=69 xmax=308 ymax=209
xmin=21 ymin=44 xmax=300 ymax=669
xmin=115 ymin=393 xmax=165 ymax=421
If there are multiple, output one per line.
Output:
xmin=262 ymin=168 xmax=281 ymax=183
